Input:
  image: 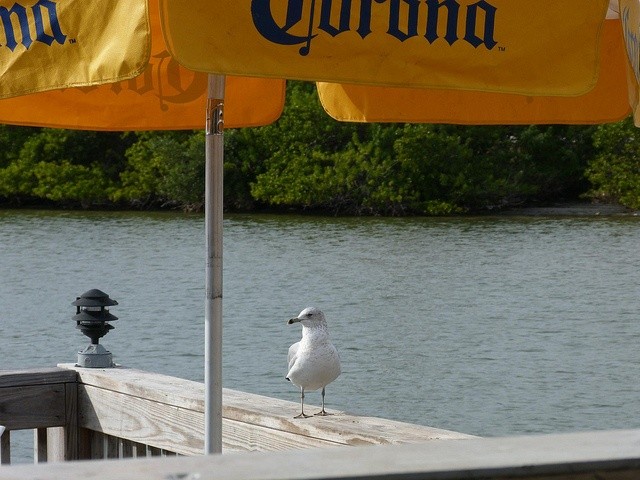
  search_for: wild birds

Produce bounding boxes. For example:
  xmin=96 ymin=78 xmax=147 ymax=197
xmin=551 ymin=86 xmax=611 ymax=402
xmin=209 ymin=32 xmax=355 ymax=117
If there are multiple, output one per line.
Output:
xmin=285 ymin=307 xmax=341 ymax=420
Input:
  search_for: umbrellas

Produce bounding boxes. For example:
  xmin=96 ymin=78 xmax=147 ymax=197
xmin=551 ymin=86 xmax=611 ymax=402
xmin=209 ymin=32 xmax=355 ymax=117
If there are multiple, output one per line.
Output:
xmin=0 ymin=0 xmax=639 ymax=456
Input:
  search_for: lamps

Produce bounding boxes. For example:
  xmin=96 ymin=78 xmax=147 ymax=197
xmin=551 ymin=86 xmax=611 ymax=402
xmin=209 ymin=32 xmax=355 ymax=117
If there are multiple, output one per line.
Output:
xmin=73 ymin=289 xmax=118 ymax=345
